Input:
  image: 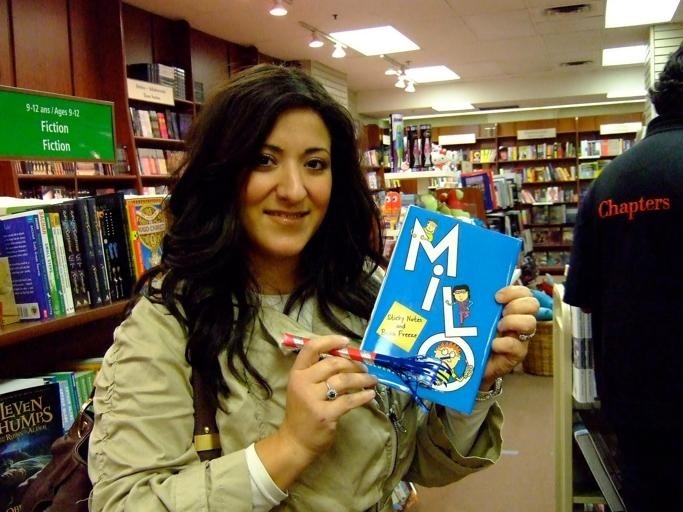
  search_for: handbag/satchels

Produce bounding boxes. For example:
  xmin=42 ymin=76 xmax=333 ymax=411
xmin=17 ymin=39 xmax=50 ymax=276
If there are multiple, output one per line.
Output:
xmin=16 ymin=293 xmax=222 ymax=511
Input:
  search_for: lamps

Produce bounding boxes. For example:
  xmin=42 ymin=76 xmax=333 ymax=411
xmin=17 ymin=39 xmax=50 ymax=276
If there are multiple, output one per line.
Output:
xmin=266 ymin=0 xmax=293 ymax=17
xmin=379 ymin=54 xmax=418 ymax=95
xmin=296 ymin=18 xmax=348 ymax=59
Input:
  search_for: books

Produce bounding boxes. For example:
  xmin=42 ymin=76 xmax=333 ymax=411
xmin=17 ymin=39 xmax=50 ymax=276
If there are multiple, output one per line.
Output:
xmin=353 ymin=114 xmax=631 ymax=264
xmin=0 ymin=149 xmax=134 ymax=326
xmin=127 ymin=62 xmax=205 ymax=280
xmin=2 ymin=358 xmax=104 ymax=464
xmin=559 ymin=299 xmax=627 ymax=512
xmin=357 ymin=202 xmax=525 ymax=419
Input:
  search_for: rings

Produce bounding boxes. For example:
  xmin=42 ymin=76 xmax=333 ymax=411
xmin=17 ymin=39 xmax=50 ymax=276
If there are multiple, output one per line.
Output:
xmin=320 ymin=378 xmax=338 ymax=399
xmin=517 ymin=327 xmax=537 ymax=343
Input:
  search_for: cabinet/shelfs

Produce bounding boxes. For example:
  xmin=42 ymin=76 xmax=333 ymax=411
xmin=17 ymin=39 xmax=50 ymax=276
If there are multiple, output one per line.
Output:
xmin=0 ymin=140 xmax=142 ymax=205
xmin=90 ymin=1 xmax=303 ymax=200
xmin=357 ymin=112 xmax=646 ymax=306
xmin=547 ymin=280 xmax=607 ymax=511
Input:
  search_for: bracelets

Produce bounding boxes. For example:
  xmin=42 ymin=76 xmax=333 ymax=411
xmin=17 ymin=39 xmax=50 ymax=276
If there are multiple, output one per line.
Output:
xmin=473 ymin=376 xmax=505 ymax=401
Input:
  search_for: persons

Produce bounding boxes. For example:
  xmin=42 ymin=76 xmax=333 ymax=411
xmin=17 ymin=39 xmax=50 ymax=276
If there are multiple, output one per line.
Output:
xmin=560 ymin=39 xmax=683 ymax=512
xmin=85 ymin=61 xmax=542 ymax=512
xmin=520 ymin=250 xmax=539 ymax=285
